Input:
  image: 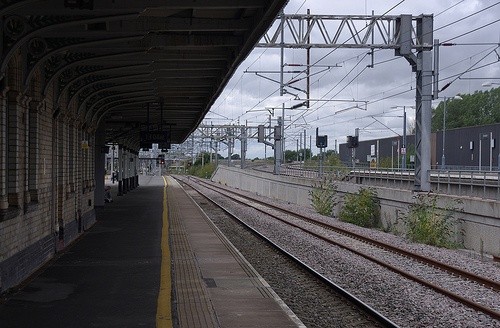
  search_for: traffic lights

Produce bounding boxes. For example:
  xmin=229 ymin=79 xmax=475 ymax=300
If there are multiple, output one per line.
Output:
xmin=317 ymin=135 xmax=326 ymax=147
xmin=162 ymin=158 xmax=166 ymax=164
